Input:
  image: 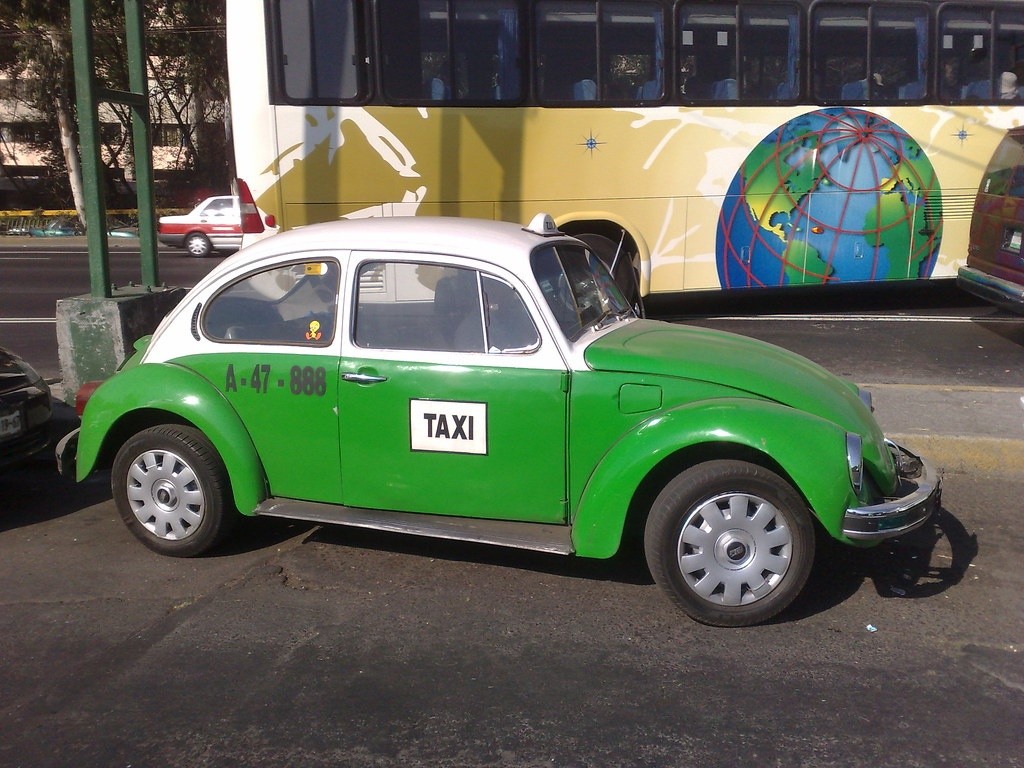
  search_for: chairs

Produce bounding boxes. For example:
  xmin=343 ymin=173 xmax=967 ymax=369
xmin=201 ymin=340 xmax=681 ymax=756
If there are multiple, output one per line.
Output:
xmin=433 ymin=276 xmax=471 ymax=350
xmin=368 ymin=72 xmax=1024 ymax=103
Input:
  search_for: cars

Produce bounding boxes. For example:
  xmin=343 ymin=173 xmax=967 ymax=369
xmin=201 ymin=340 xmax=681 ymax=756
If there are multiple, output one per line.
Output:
xmin=956 ymin=126 xmax=1024 ymax=316
xmin=29 ymin=220 xmax=138 ymax=238
xmin=0 ymin=346 xmax=55 ymax=463
xmin=55 ymin=215 xmax=944 ymax=628
xmin=158 ymin=196 xmax=243 ymax=258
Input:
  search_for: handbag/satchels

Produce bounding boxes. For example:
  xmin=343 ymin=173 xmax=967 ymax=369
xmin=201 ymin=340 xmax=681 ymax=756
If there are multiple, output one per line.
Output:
xmin=270 ymin=274 xmax=334 ymax=323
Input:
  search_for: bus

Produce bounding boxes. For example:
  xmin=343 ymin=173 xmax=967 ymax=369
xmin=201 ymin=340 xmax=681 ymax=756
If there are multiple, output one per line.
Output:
xmin=225 ymin=0 xmax=1024 ymax=320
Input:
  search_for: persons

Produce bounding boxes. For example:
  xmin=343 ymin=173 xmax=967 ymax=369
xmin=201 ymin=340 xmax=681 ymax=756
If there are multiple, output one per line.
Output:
xmin=989 ymin=72 xmax=1020 ymax=99
xmin=453 ymin=274 xmax=515 ymax=351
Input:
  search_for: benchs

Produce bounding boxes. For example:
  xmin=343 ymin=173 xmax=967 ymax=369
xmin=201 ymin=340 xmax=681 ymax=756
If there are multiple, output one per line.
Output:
xmin=226 ymin=308 xmax=337 ymax=344
